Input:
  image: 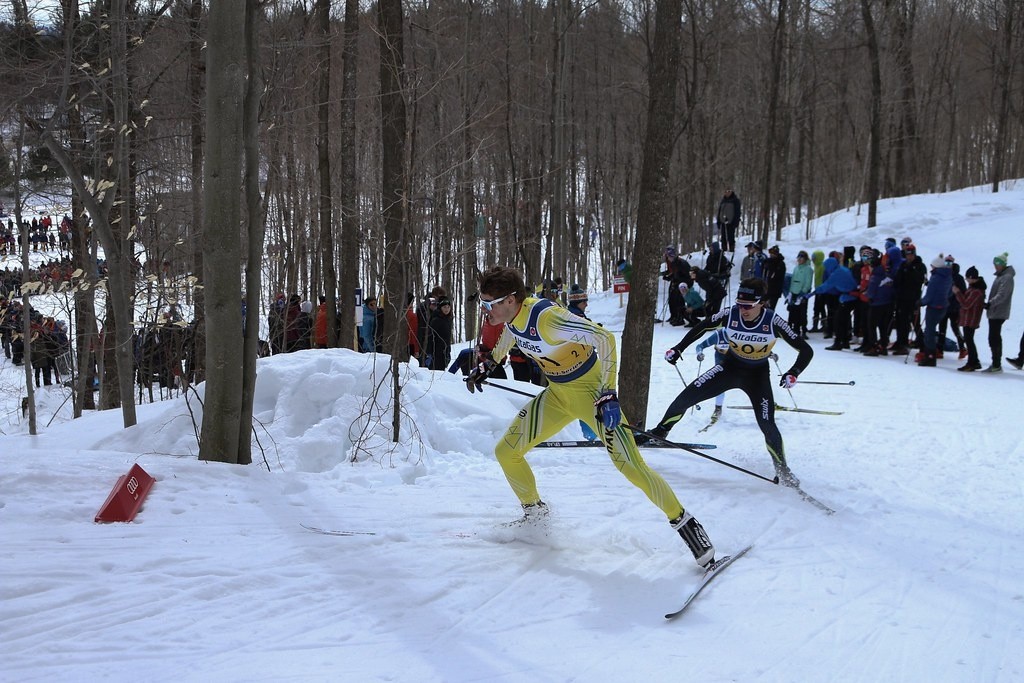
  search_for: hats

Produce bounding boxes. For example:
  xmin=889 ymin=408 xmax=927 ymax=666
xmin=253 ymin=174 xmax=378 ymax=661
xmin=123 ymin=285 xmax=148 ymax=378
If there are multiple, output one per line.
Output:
xmin=830 ymin=236 xmax=917 ymax=263
xmin=992 ymin=252 xmax=1009 ymax=265
xmin=436 ymin=296 xmax=450 ymax=308
xmin=568 ymin=284 xmax=589 ymax=305
xmin=966 ymin=266 xmax=978 ymax=278
xmin=744 ymin=241 xmax=782 ymax=255
xmin=930 ymin=254 xmax=953 ymax=269
xmin=665 ymin=245 xmax=701 ymax=291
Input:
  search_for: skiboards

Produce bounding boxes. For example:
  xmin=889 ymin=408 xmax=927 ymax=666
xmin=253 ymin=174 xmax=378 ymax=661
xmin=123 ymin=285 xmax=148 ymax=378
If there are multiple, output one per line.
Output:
xmin=533 ymin=441 xmax=841 ymax=515
xmin=697 ymin=405 xmax=847 ymax=434
xmin=300 ymin=521 xmax=762 ymax=619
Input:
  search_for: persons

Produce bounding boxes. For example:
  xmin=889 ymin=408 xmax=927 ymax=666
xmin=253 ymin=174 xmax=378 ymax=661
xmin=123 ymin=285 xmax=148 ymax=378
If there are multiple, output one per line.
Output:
xmin=481 ymin=278 xmax=596 ymax=441
xmin=717 ymin=185 xmax=741 ymax=253
xmin=635 ymin=276 xmax=814 ymax=487
xmin=268 ymin=294 xmax=327 ymax=356
xmin=0 ymin=258 xmax=61 ymax=298
xmin=784 ymin=238 xmax=905 ymax=356
xmin=59 ymin=214 xmax=92 ymax=250
xmin=358 ymin=295 xmax=384 ymax=353
xmin=659 ymin=241 xmax=733 ymax=328
xmin=466 ymin=266 xmax=714 ymax=567
xmin=739 ymin=240 xmax=786 ymax=311
xmin=135 ymin=258 xmax=145 ymax=277
xmin=888 ymin=237 xmax=1024 ymax=373
xmin=0 ymin=296 xmax=69 ymax=387
xmin=408 ymin=287 xmax=454 ymax=371
xmin=62 ymin=253 xmax=107 ymax=281
xmin=0 ymin=215 xmax=56 ymax=256
xmin=133 ymin=313 xmax=194 ymax=388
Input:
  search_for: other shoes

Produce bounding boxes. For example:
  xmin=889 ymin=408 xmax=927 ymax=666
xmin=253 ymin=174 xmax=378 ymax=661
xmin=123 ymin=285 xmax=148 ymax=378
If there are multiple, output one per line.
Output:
xmin=670 ymin=509 xmax=716 ymax=568
xmin=852 ymin=333 xmax=943 ymax=368
xmin=981 ymin=366 xmax=1003 ymax=373
xmin=495 ymin=500 xmax=553 ymax=530
xmin=1006 ymin=357 xmax=1022 ymax=370
xmin=800 ymin=326 xmax=832 ymax=339
xmin=957 ymin=365 xmax=982 ymax=371
xmin=824 ymin=341 xmax=850 ymax=351
xmin=958 ymin=349 xmax=967 ymax=361
xmin=631 ymin=424 xmax=671 ymax=447
xmin=774 ymin=460 xmax=801 ymax=487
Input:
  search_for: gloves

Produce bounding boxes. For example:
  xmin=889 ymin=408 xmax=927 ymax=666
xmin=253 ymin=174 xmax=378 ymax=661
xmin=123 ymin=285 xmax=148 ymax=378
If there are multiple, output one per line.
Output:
xmin=780 ymin=371 xmax=797 ymax=390
xmin=665 ymin=347 xmax=679 ymax=365
xmin=594 ymin=394 xmax=621 ymax=431
xmin=466 ymin=362 xmax=492 ymax=393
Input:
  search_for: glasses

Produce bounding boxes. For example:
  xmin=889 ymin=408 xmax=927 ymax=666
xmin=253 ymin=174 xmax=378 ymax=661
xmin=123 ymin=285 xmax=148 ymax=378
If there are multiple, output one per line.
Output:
xmin=544 ymin=288 xmax=558 ymax=293
xmin=479 ymin=289 xmax=516 ymax=311
